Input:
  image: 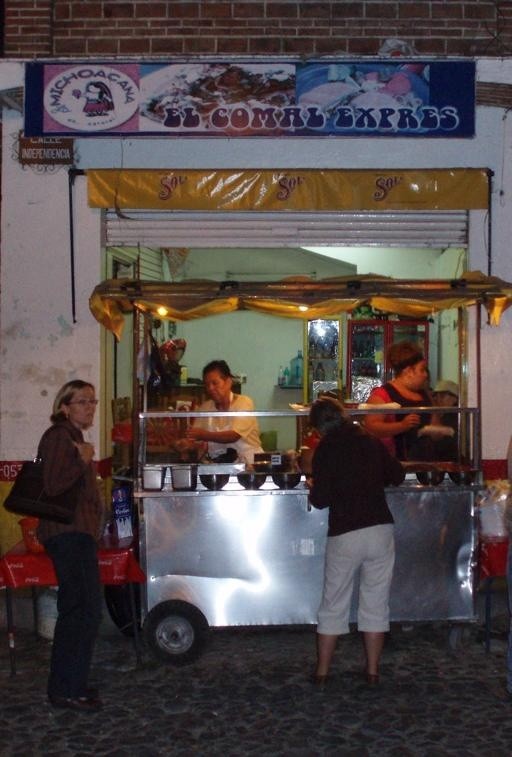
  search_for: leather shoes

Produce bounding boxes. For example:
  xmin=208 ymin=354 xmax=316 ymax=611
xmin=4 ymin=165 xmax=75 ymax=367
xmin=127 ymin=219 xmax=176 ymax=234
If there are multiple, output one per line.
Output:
xmin=77 ymin=688 xmax=98 ymax=697
xmin=52 ymin=696 xmax=102 ymax=712
xmin=312 ymin=676 xmax=329 ymax=692
xmin=364 ymin=675 xmax=379 ymax=684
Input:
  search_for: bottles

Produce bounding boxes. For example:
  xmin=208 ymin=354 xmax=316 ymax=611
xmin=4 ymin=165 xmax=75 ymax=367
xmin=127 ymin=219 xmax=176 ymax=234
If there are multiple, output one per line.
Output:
xmin=277 ymin=366 xmax=290 ymax=386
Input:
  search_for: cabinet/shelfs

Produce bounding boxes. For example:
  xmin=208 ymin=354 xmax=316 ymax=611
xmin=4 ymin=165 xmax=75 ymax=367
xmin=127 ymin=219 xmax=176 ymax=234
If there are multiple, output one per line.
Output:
xmin=140 ymin=379 xmax=242 ymax=413
xmin=348 ymin=321 xmax=384 ymax=399
xmin=385 ymin=315 xmax=430 ymax=382
xmin=304 ymin=314 xmax=347 ymax=406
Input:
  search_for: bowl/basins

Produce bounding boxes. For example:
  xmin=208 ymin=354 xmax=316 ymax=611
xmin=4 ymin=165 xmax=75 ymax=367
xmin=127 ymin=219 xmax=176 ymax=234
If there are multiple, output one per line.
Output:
xmin=199 ymin=473 xmax=230 ymax=492
xmin=271 ymin=471 xmax=301 ymax=491
xmin=447 ymin=469 xmax=478 ymax=488
xmin=416 ymin=470 xmax=446 ymax=487
xmin=236 ymin=471 xmax=266 ymax=491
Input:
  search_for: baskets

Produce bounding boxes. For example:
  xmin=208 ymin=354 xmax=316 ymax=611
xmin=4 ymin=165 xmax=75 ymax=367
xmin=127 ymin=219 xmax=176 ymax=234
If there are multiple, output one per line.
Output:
xmin=18 ymin=519 xmax=44 ymax=553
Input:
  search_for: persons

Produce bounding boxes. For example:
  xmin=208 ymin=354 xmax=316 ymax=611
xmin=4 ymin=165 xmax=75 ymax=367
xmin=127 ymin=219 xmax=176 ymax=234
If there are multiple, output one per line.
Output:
xmin=361 ymin=340 xmax=447 ymax=465
xmin=32 ymin=378 xmax=103 ymax=712
xmin=176 ymin=359 xmax=267 ymax=465
xmin=305 ymin=395 xmax=407 ymax=690
xmin=431 ymin=379 xmax=459 ymax=452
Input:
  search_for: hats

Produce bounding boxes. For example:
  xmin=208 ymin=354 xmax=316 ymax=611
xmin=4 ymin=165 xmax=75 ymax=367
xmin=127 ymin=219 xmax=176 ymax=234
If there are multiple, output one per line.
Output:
xmin=433 ymin=380 xmax=458 ymax=398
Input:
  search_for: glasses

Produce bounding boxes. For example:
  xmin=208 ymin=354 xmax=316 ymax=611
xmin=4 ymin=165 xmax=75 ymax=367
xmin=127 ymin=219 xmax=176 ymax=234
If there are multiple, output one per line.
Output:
xmin=65 ymin=400 xmax=97 ymax=406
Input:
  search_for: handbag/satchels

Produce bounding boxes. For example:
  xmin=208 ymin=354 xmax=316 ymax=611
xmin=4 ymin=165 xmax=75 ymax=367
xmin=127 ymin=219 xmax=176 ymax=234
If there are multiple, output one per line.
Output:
xmin=149 ymin=332 xmax=167 ymax=391
xmin=3 ymin=461 xmax=82 ymax=524
